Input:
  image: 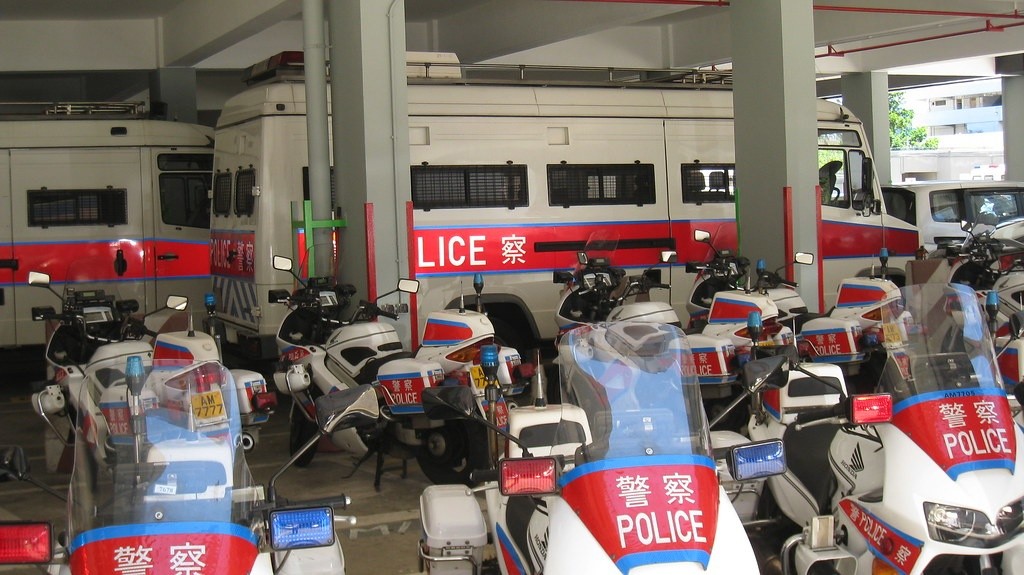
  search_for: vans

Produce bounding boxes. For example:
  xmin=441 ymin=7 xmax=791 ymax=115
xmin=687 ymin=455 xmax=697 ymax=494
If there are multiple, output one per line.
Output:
xmin=877 ymin=179 xmax=1024 ymax=246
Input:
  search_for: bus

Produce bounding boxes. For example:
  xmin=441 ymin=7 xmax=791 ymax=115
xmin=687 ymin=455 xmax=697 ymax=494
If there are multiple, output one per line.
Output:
xmin=0 ymin=99 xmax=225 ymax=350
xmin=207 ymin=51 xmax=920 ymax=379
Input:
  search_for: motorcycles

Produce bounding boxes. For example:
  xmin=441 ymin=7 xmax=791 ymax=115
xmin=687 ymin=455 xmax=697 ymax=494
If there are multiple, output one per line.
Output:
xmin=0 ymin=218 xmax=1024 ymax=575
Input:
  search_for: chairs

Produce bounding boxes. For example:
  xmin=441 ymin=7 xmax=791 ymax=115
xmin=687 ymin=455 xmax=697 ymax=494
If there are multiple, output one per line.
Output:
xmin=690 ymin=171 xmax=726 ymax=201
